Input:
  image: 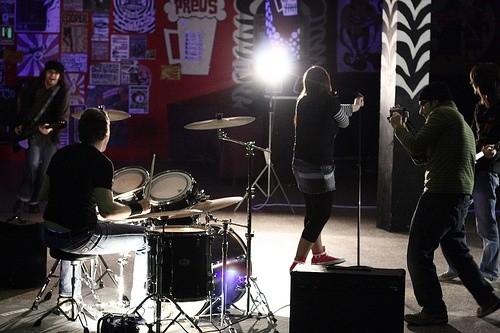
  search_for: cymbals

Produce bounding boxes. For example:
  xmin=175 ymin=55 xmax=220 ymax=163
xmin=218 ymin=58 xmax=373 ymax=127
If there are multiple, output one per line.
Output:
xmin=184 ymin=116 xmax=255 ymax=130
xmin=71 ymin=108 xmax=131 ymax=121
xmin=126 ymin=208 xmax=203 ymax=220
xmin=168 ymin=196 xmax=243 ymax=220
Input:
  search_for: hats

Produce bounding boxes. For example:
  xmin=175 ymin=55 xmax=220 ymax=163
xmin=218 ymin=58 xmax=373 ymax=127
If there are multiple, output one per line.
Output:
xmin=418 ymin=82 xmax=451 ymax=100
xmin=44 ymin=60 xmax=65 ymax=74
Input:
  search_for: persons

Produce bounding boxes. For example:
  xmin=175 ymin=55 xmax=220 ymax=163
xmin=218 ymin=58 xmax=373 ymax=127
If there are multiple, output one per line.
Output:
xmin=40 ymin=108 xmax=170 ymax=324
xmin=389 ymin=83 xmax=500 ymax=326
xmin=14 ymin=60 xmax=70 ymax=214
xmin=435 ymin=64 xmax=500 ymax=284
xmin=289 ymin=67 xmax=363 ymax=272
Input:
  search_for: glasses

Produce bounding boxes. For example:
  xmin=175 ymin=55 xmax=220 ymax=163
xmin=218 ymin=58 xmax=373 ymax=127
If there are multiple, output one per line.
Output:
xmin=418 ymin=98 xmax=431 ymax=105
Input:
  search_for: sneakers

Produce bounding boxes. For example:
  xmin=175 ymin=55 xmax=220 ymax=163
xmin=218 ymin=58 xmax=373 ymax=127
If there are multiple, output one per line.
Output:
xmin=290 ymin=260 xmax=305 ymax=271
xmin=311 ymin=246 xmax=346 ymax=265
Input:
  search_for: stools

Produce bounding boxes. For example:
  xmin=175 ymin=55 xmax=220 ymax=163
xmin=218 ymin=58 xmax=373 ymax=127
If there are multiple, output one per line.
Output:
xmin=35 ymin=247 xmax=98 ymax=333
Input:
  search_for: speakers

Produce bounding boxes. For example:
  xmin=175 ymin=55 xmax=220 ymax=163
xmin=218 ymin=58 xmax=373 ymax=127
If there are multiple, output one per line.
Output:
xmin=289 ymin=263 xmax=405 ymax=333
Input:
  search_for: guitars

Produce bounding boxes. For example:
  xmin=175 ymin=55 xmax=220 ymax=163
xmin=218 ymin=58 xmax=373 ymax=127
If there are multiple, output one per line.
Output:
xmin=475 ymin=141 xmax=500 ymax=160
xmin=8 ymin=113 xmax=67 ymax=153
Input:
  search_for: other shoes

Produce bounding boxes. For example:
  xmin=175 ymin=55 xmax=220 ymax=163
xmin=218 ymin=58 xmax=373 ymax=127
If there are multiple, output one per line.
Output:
xmin=13 ymin=199 xmax=41 ymax=216
xmin=477 ymin=294 xmax=500 ymax=317
xmin=405 ymin=301 xmax=448 ymax=324
xmin=437 ymin=272 xmax=457 ymax=281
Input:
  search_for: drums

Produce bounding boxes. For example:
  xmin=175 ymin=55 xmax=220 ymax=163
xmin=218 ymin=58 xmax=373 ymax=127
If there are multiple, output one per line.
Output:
xmin=143 ymin=170 xmax=201 ymax=212
xmin=211 ymin=224 xmax=252 ymax=308
xmin=113 ymin=167 xmax=150 ymax=203
xmin=146 ymin=226 xmax=213 ymax=302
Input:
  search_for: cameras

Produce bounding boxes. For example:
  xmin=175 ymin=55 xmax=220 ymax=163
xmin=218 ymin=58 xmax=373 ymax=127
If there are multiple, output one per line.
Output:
xmin=387 ymin=107 xmax=409 ymax=124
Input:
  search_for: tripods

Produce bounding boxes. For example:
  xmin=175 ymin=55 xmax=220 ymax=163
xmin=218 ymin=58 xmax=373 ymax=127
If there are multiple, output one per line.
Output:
xmin=130 ymin=129 xmax=279 ymax=333
